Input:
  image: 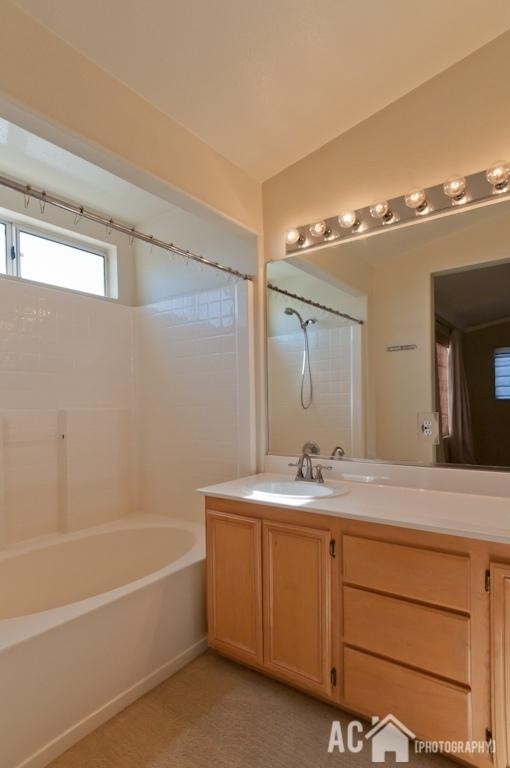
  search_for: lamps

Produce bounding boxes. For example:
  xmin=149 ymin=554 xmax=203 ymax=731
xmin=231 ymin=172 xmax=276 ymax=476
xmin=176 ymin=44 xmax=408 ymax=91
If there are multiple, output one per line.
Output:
xmin=283 ymin=159 xmax=510 ymax=256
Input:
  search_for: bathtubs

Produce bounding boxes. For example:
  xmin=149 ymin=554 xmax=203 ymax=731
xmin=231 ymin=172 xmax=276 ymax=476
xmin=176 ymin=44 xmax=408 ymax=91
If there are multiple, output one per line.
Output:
xmin=0 ymin=519 xmax=206 ymax=766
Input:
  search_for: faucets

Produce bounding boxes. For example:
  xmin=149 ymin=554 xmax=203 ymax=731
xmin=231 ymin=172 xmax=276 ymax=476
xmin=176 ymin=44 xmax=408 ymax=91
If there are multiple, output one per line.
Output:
xmin=330 ymin=445 xmax=347 ymax=458
xmin=298 ymin=453 xmax=312 ymax=480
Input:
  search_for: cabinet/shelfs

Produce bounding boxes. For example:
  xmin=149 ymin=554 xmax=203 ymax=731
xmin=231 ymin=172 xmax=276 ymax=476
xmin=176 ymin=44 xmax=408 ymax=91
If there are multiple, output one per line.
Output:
xmin=204 ymin=496 xmax=510 ymax=768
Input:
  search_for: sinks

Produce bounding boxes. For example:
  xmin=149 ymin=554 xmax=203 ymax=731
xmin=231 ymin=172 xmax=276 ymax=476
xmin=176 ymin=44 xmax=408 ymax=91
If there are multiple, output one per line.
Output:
xmin=253 ymin=480 xmax=332 ymax=500
